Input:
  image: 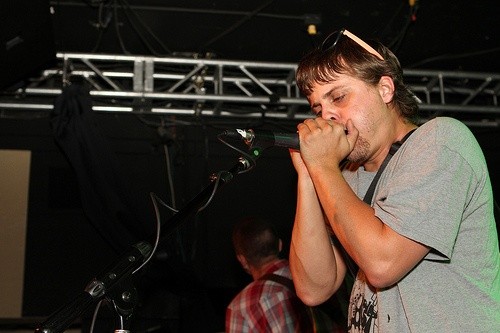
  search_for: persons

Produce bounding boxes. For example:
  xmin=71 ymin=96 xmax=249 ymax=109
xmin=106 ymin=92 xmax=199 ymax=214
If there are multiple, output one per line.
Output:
xmin=224 ymin=217 xmax=340 ymax=333
xmin=287 ymin=38 xmax=499 ymax=333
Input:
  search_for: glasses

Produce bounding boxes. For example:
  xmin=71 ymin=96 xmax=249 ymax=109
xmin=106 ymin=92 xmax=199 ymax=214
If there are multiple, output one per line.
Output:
xmin=303 ymin=28 xmax=385 ymax=64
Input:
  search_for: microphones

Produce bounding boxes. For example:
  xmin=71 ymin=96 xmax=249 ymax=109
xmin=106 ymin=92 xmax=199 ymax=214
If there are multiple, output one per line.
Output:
xmin=223 ymin=128 xmax=300 ymax=151
